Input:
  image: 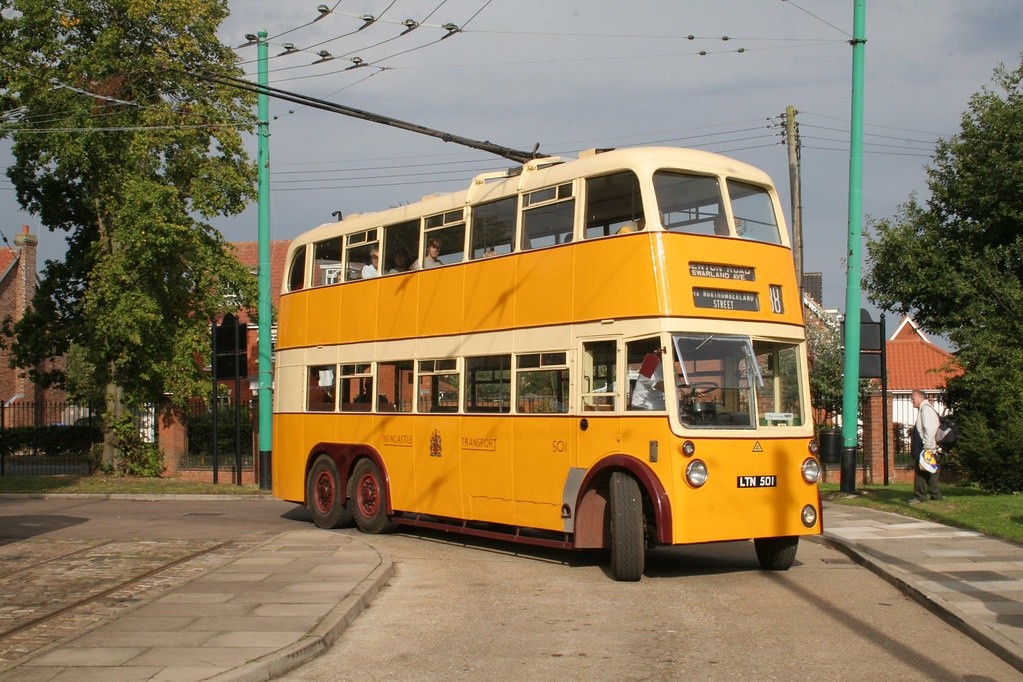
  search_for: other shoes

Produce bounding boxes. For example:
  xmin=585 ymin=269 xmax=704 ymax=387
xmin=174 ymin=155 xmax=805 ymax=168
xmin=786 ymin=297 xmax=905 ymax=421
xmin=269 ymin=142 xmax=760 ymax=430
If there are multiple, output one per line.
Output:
xmin=907 ymin=497 xmax=920 ymax=504
xmin=929 ymin=497 xmax=937 ymax=501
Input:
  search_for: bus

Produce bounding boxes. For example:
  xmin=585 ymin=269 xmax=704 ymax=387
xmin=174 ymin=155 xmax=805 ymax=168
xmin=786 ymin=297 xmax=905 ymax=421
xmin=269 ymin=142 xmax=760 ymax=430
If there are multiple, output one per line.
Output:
xmin=269 ymin=147 xmax=825 ymax=583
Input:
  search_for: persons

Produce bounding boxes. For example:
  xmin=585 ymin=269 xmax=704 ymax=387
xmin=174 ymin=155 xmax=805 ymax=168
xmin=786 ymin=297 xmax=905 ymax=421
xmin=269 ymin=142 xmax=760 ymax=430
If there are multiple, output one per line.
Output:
xmin=714 ymin=195 xmax=741 ymax=236
xmin=337 ymin=272 xmax=341 ymax=282
xmin=424 ymin=238 xmax=442 ymax=267
xmin=362 ymin=249 xmax=379 ymax=278
xmin=631 ymin=340 xmax=691 ymax=410
xmin=354 ymin=377 xmax=388 ymax=403
xmin=327 ymin=379 xmax=335 ymax=402
xmin=906 ymin=390 xmax=943 ymax=505
xmin=388 ymin=249 xmax=409 ymax=274
xmin=615 ymin=207 xmax=665 ymax=235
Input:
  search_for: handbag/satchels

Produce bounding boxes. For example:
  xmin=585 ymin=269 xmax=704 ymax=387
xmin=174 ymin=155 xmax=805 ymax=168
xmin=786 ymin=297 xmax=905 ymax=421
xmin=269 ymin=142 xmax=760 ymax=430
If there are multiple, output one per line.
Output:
xmin=934 ymin=420 xmax=957 ymax=451
